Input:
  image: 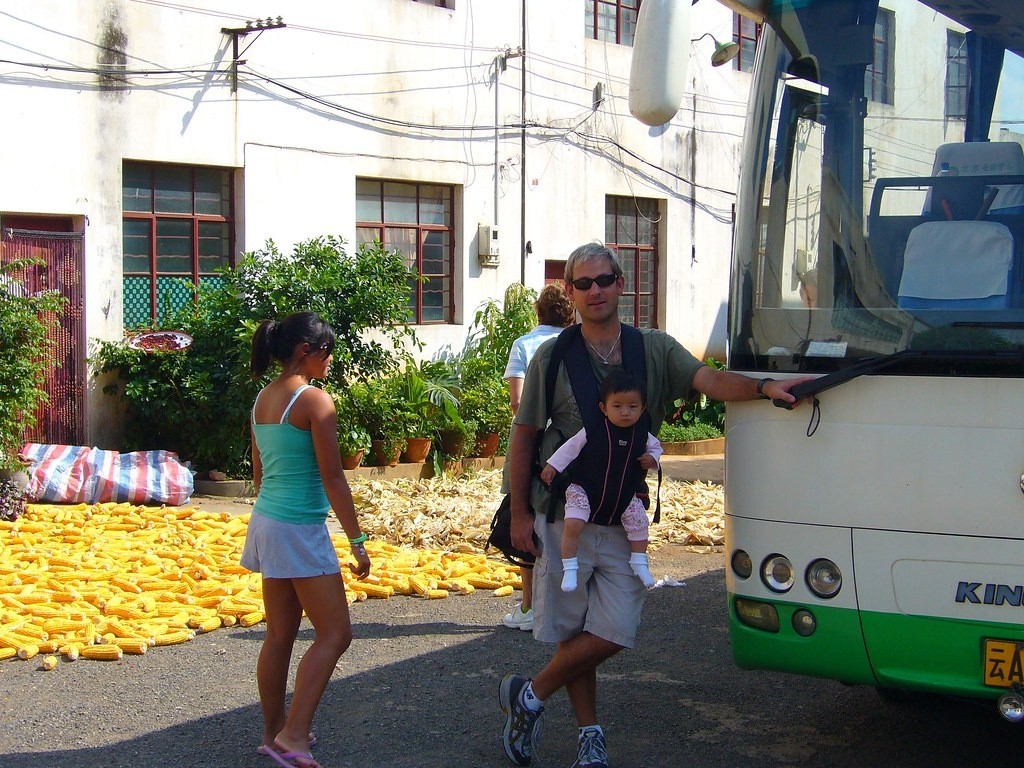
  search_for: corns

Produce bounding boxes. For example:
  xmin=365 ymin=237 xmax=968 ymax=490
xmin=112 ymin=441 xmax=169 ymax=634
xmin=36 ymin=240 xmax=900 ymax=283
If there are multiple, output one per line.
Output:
xmin=0 ymin=501 xmax=524 ymax=670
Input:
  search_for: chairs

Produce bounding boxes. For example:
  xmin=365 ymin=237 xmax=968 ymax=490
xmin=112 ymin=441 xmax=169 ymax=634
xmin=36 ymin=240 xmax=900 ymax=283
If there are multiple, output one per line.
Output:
xmin=897 ymin=220 xmax=1015 ymax=313
xmin=921 ymin=142 xmax=1024 ymax=216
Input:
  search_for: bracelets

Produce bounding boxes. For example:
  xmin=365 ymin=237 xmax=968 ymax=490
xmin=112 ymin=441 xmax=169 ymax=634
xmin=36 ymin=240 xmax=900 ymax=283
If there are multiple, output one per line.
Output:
xmin=757 ymin=378 xmax=775 ymax=400
xmin=349 ymin=532 xmax=367 ymax=544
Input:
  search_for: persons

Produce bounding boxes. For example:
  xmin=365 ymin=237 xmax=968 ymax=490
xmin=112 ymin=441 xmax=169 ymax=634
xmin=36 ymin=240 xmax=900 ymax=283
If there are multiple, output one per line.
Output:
xmin=500 ymin=284 xmax=576 ymax=631
xmin=500 ymin=243 xmax=815 ymax=768
xmin=241 ymin=312 xmax=370 ymax=768
xmin=800 ymin=269 xmax=818 ymax=308
xmin=541 ymin=367 xmax=663 ymax=592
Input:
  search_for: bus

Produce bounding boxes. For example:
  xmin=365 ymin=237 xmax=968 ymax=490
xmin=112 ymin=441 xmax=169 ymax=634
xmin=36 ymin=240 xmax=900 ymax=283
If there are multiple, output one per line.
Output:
xmin=627 ymin=0 xmax=1024 ymax=724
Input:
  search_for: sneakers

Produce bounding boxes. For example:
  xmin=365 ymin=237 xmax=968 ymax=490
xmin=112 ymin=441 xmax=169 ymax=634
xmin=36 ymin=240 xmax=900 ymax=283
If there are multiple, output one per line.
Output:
xmin=499 ymin=674 xmax=545 ymax=767
xmin=504 ymin=602 xmax=534 ymax=630
xmin=571 ymin=727 xmax=609 ymax=768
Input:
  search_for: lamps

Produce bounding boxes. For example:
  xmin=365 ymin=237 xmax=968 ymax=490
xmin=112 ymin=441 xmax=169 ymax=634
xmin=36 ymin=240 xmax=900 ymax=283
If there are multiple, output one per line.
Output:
xmin=691 ymin=33 xmax=740 ymax=66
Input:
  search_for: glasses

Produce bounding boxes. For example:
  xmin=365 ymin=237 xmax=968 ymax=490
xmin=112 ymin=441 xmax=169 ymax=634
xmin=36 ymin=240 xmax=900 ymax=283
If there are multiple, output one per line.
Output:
xmin=572 ymin=274 xmax=618 ymax=290
xmin=309 ymin=342 xmax=334 ymax=353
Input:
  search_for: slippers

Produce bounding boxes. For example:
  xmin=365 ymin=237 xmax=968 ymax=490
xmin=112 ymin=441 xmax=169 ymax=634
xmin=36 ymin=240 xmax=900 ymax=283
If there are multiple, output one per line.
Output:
xmin=263 ymin=744 xmax=313 ymax=768
xmin=257 ymin=732 xmax=317 ymax=755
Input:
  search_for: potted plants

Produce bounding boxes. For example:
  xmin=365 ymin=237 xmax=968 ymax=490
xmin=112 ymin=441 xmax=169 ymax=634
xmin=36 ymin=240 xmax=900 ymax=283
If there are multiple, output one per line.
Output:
xmin=334 ymin=358 xmax=509 ymax=476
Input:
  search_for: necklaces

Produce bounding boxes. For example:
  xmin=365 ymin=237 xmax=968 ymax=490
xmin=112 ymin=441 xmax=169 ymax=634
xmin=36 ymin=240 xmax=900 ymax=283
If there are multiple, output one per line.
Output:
xmin=581 ymin=322 xmax=621 ymax=364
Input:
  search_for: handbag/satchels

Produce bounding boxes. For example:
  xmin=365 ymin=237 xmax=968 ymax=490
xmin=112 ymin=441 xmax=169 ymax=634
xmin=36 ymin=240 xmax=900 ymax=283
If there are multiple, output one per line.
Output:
xmin=484 ymin=492 xmax=539 ymax=569
